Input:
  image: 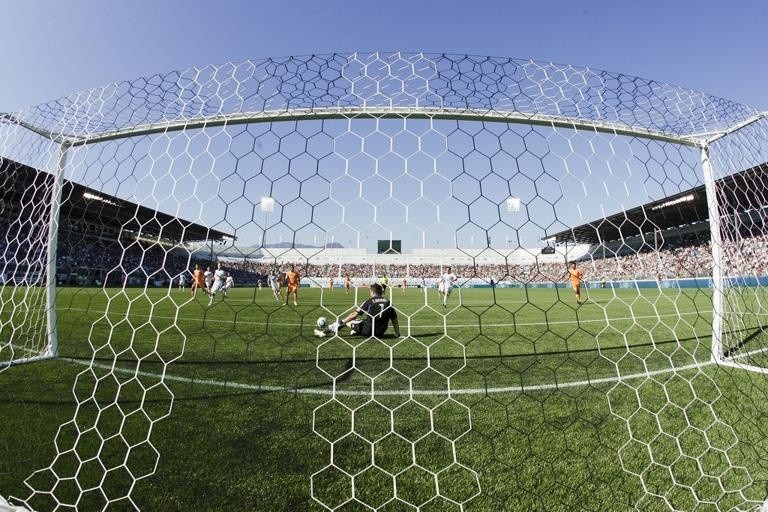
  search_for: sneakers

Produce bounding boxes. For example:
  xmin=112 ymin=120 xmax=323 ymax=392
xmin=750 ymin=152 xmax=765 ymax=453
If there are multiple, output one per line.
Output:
xmin=208 ymin=302 xmax=212 ymax=306
xmin=313 ymin=328 xmax=326 ymax=338
xmin=282 ymin=303 xmax=288 ymax=306
xmin=294 ymin=301 xmax=298 ymax=306
xmin=350 ymin=330 xmax=357 ymax=336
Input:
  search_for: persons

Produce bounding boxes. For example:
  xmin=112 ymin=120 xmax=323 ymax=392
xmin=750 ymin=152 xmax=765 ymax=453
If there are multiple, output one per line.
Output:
xmin=313 ymin=283 xmax=400 ymax=339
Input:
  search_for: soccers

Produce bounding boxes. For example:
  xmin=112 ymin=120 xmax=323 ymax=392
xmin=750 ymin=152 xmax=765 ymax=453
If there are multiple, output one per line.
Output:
xmin=317 ymin=317 xmax=328 ymax=328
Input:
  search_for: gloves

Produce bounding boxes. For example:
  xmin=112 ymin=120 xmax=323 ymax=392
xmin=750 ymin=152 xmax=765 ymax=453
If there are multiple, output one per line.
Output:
xmin=328 ymin=321 xmax=344 ymax=332
xmin=395 ymin=333 xmax=406 ymax=338
